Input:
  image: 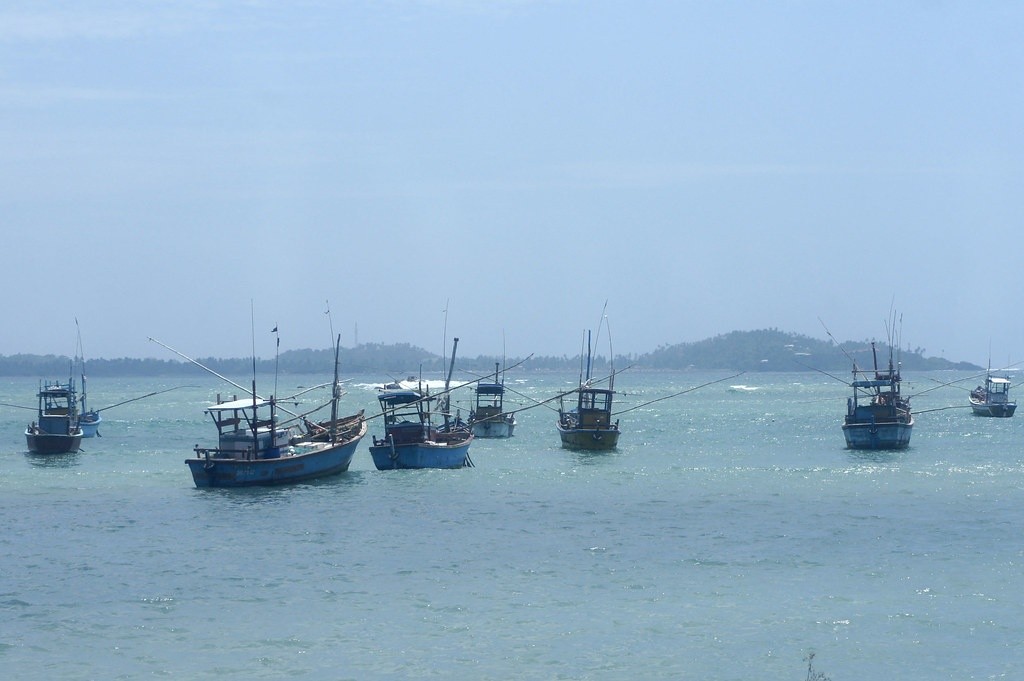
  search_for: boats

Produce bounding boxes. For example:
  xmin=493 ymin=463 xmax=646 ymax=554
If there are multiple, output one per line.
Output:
xmin=967 ymin=377 xmax=1018 ymax=419
xmin=24 ymin=377 xmax=83 ymax=455
xmin=369 ymin=388 xmax=477 ymax=471
xmin=184 ymin=297 xmax=366 ymax=489
xmin=840 ymin=342 xmax=915 ymax=449
xmin=468 ymin=381 xmax=517 ymax=438
xmin=72 ymin=318 xmax=100 ymax=438
xmin=557 ymin=386 xmax=621 ymax=452
